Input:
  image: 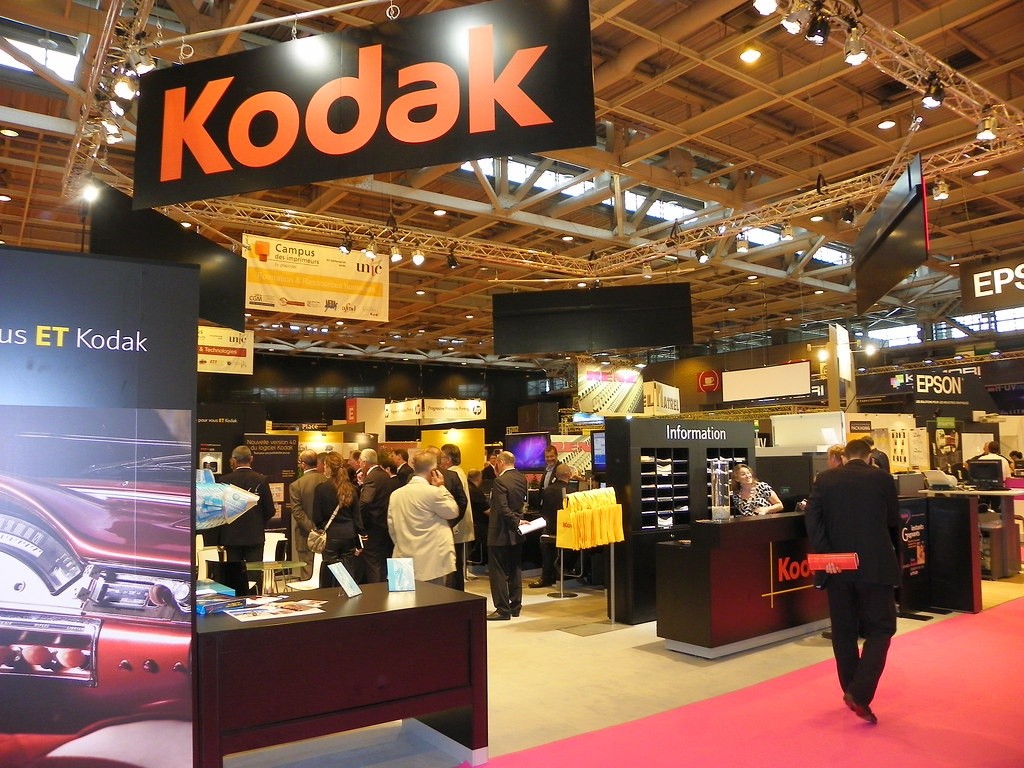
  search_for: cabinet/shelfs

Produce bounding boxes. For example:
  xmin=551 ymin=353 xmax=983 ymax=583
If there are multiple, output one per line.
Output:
xmin=603 ymin=417 xmax=755 ymax=626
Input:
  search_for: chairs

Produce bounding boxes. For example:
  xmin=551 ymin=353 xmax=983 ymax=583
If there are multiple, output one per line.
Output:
xmin=263 ymin=540 xmax=290 ymax=594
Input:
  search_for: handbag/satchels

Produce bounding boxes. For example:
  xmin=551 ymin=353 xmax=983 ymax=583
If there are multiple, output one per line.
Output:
xmin=308 ymin=530 xmax=327 ymax=553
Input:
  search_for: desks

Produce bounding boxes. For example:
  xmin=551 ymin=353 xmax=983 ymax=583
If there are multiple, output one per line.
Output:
xmin=246 ymin=560 xmax=306 ymax=592
xmin=918 ymin=488 xmax=1024 ymax=576
xmin=197 ymin=580 xmax=488 ymax=768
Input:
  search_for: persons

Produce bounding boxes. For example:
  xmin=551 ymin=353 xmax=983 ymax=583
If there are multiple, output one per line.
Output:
xmin=215 ymin=444 xmax=277 ymax=594
xmin=288 ymin=447 xmax=393 ymax=588
xmin=1009 ymin=450 xmax=1023 ymax=460
xmin=375 ymin=444 xmax=475 ymax=592
xmin=732 ymin=464 xmax=784 ymax=516
xmin=528 ymin=444 xmax=562 ymax=587
xmin=539 ymin=462 xmax=591 ymax=580
xmin=963 ymin=441 xmax=1014 ymax=511
xmin=482 ymin=453 xmax=500 ymax=487
xmin=795 ymin=435 xmax=903 ymax=722
xmin=487 ymin=451 xmax=531 ymax=620
xmin=467 ymin=468 xmax=491 ymax=575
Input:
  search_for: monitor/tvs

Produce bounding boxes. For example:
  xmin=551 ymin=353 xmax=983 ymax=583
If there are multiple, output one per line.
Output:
xmin=968 ymin=459 xmax=1003 ymax=490
xmin=590 ymin=430 xmax=606 ymax=472
xmin=505 ymin=432 xmax=551 ymax=474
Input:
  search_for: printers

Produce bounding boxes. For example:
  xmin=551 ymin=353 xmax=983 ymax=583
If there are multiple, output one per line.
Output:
xmin=925 ymin=475 xmax=957 ymax=490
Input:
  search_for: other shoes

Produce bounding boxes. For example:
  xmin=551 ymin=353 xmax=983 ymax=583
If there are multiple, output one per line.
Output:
xmin=528 ymin=579 xmax=553 ymax=588
xmin=510 ymin=611 xmax=519 ymax=617
xmin=843 ymin=692 xmax=877 ymax=724
xmin=487 ymin=611 xmax=510 ymax=620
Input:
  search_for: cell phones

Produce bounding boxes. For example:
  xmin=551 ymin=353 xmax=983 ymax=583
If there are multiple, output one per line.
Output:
xmin=431 ymin=468 xmax=438 ymax=477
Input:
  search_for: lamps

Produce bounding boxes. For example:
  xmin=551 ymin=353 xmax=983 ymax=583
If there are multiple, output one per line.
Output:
xmin=109 ymin=0 xmax=997 ymax=278
